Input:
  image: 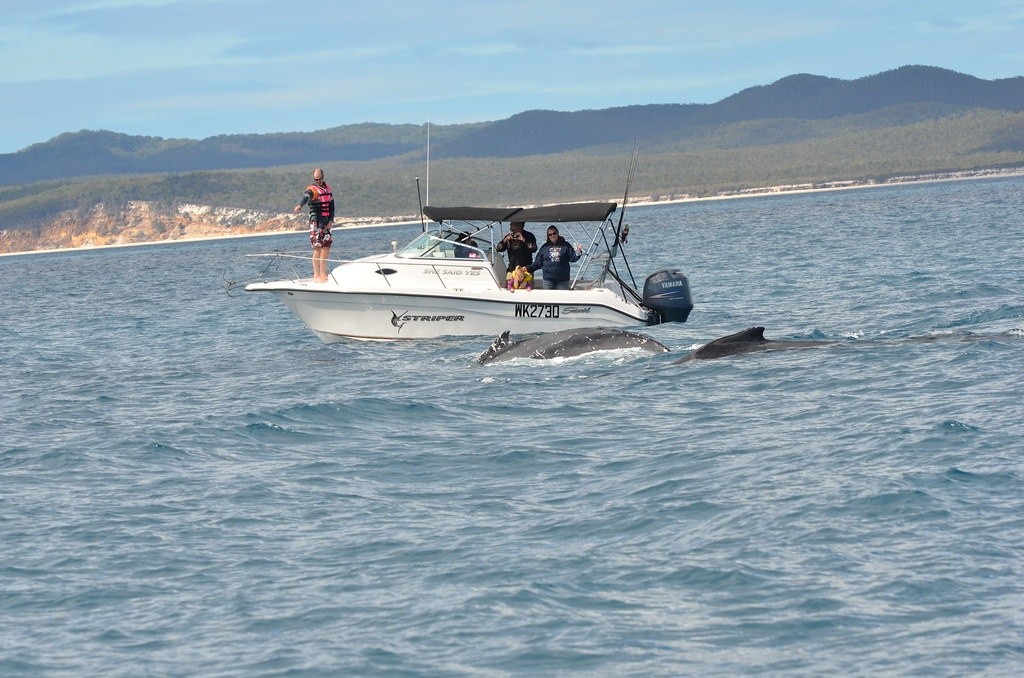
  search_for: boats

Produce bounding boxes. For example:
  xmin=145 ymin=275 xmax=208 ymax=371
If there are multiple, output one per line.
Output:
xmin=242 ymin=120 xmax=694 ymax=348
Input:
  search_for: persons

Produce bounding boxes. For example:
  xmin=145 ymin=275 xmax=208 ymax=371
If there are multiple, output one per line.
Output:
xmin=295 ymin=169 xmax=335 ymax=282
xmin=453 ymin=231 xmax=480 ymax=258
xmin=523 ymin=225 xmax=583 ymax=290
xmin=495 ymin=222 xmax=538 ymax=289
xmin=507 ymin=265 xmax=533 ymax=293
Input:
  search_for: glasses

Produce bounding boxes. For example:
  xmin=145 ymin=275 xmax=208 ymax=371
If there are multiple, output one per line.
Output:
xmin=549 ymin=232 xmax=558 ymax=236
xmin=314 ymin=177 xmax=323 ymax=181
